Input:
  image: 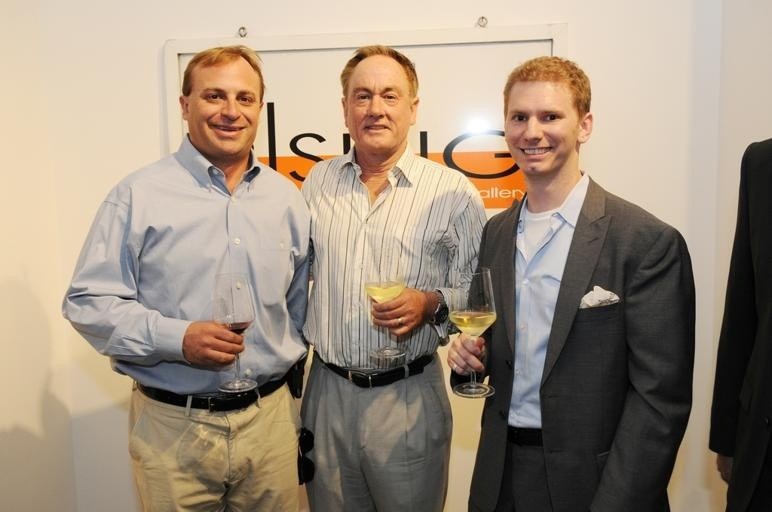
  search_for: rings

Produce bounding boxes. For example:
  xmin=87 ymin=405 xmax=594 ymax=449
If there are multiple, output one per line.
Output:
xmin=396 ymin=317 xmax=403 ymax=326
xmin=452 ymin=363 xmax=459 ymax=375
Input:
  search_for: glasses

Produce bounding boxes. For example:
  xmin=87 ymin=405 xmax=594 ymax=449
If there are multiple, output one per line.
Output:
xmin=296 ymin=426 xmax=314 ymax=485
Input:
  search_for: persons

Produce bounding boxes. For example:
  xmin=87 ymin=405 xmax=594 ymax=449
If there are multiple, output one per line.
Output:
xmin=446 ymin=56 xmax=695 ymax=512
xmin=709 ymin=139 xmax=770 ymax=512
xmin=59 ymin=43 xmax=311 ymax=512
xmin=301 ymin=46 xmax=488 ymax=511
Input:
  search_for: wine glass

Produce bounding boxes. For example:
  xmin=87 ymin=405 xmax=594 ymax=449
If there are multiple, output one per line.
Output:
xmin=444 ymin=264 xmax=501 ymax=401
xmin=361 ymin=241 xmax=409 ymax=361
xmin=209 ymin=269 xmax=260 ymax=394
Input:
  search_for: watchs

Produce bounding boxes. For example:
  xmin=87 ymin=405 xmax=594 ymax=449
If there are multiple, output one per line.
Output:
xmin=428 ymin=290 xmax=449 ymax=327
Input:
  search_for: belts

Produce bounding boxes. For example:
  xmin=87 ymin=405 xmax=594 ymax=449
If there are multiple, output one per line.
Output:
xmin=508 ymin=424 xmax=542 ymax=448
xmin=136 ymin=377 xmax=288 ymax=413
xmin=315 ymin=351 xmax=435 ymax=389
